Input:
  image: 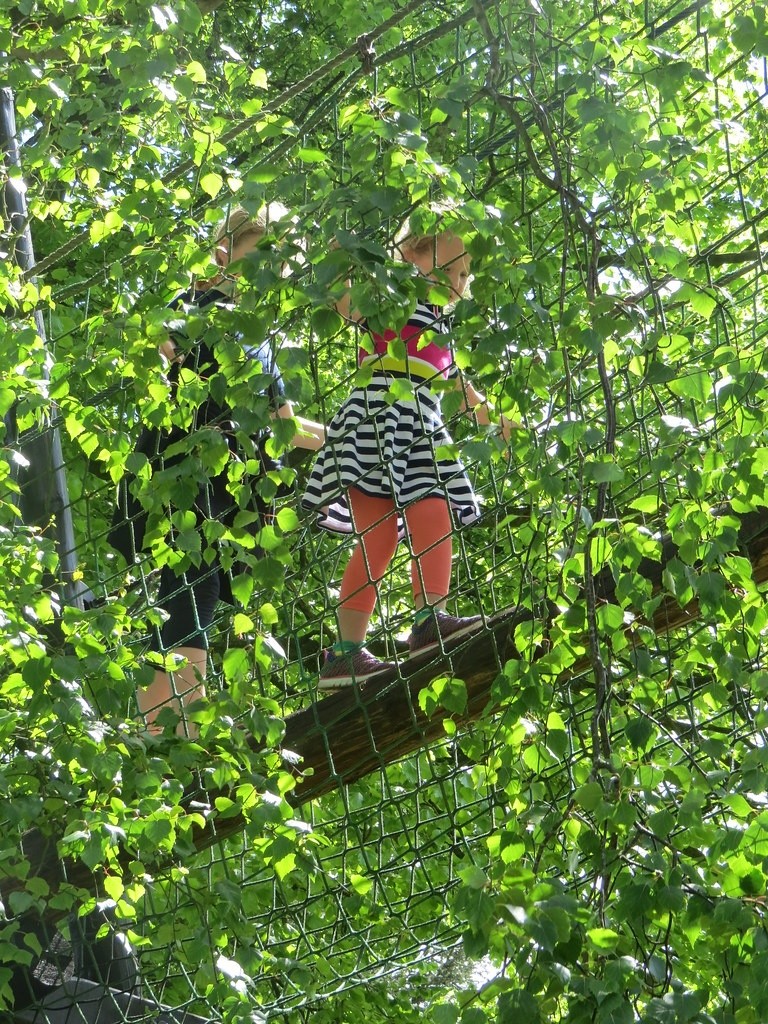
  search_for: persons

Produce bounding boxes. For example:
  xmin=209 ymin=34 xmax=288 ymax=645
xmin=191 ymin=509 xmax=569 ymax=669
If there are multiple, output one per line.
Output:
xmin=105 ymin=201 xmax=332 ymax=741
xmin=301 ymin=202 xmax=522 ymax=692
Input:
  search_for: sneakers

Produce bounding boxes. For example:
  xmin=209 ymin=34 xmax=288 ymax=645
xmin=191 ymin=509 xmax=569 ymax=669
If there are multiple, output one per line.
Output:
xmin=407 ymin=611 xmax=489 ymax=659
xmin=317 ymin=647 xmax=399 ymax=688
